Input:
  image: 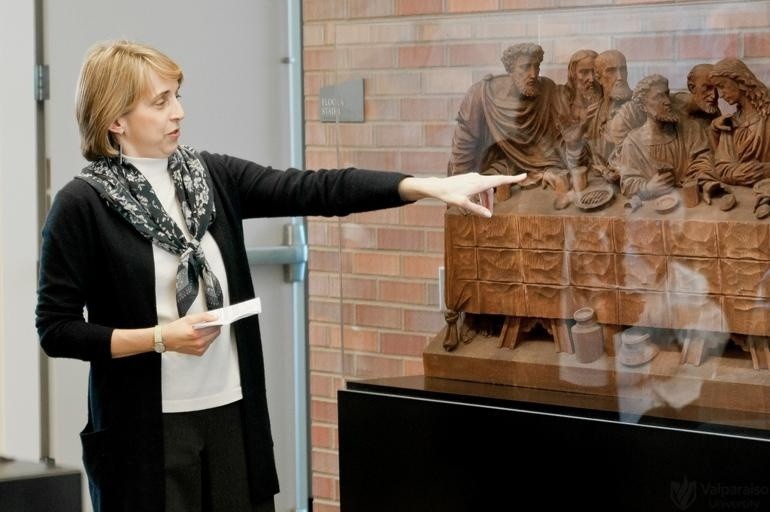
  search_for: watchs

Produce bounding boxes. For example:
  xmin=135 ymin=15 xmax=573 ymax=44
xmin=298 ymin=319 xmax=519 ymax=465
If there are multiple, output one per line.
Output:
xmin=152 ymin=324 xmax=166 ymax=354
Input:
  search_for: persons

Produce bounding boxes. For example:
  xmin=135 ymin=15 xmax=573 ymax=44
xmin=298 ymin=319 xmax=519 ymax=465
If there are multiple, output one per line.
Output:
xmin=553 ymin=49 xmax=769 ymax=204
xmin=449 ymin=41 xmax=559 ymax=205
xmin=33 ymin=40 xmax=529 ymax=512
